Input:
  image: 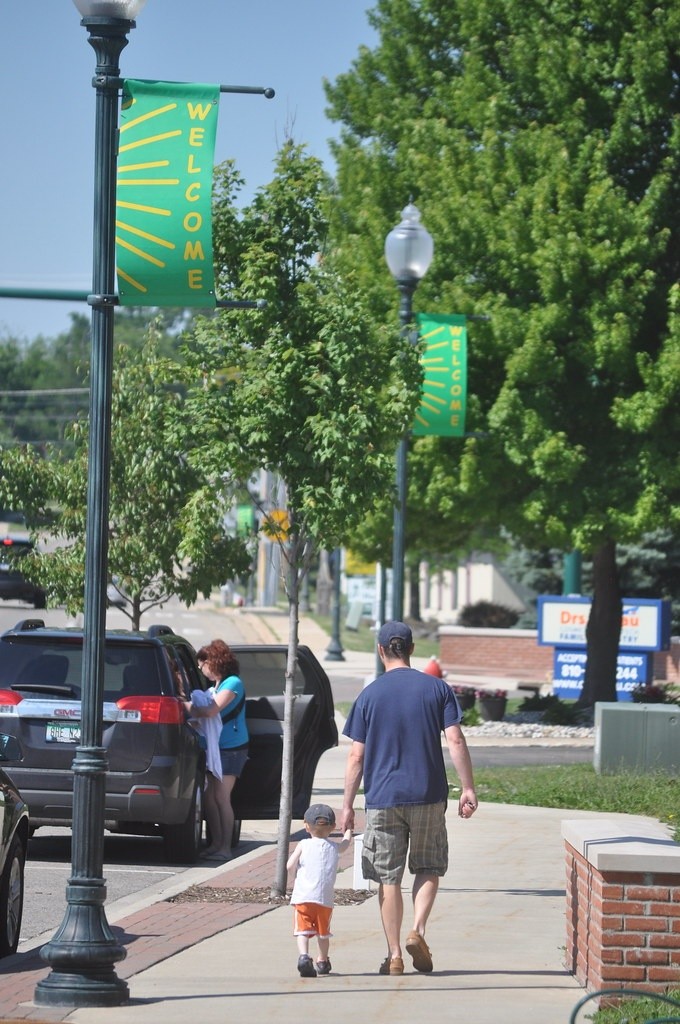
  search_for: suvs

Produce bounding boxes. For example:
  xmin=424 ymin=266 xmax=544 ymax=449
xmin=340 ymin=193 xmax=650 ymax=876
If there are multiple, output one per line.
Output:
xmin=0 ymin=767 xmax=30 ymax=959
xmin=0 ymin=620 xmax=341 ymax=859
xmin=0 ymin=534 xmax=47 ymax=609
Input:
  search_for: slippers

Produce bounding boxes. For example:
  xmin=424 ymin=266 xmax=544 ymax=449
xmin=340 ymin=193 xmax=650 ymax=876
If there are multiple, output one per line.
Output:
xmin=206 ymin=851 xmax=233 ymax=861
xmin=198 ymin=848 xmax=214 ymax=858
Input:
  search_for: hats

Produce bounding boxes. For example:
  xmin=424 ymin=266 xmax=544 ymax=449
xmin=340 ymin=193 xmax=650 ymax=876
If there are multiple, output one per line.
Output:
xmin=305 ymin=804 xmax=335 ymax=825
xmin=379 ymin=620 xmax=413 ymax=647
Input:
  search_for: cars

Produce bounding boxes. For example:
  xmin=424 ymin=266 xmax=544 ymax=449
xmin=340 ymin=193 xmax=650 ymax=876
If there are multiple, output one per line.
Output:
xmin=105 ymin=572 xmax=128 ymax=606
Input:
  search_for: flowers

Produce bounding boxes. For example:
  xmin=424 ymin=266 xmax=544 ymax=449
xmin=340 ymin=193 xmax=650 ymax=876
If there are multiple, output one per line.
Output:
xmin=452 ymin=686 xmax=476 ymax=696
xmin=475 ymin=689 xmax=507 ymax=699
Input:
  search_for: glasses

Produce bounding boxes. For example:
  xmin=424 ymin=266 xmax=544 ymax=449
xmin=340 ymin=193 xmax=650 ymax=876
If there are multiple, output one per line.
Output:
xmin=198 ymin=662 xmax=206 ymax=671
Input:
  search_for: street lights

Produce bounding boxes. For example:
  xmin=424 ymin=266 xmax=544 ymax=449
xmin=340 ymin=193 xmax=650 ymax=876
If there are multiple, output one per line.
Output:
xmin=375 ymin=189 xmax=491 ymax=682
xmin=33 ymin=0 xmax=276 ymax=1008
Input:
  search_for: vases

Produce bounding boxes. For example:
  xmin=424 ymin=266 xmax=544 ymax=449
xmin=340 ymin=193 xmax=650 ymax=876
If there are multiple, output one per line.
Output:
xmin=456 ymin=694 xmax=476 ymax=712
xmin=479 ymin=698 xmax=508 ymax=721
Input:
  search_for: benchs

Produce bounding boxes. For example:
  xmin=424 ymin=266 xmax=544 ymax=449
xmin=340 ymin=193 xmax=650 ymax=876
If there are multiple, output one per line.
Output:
xmin=517 ymin=681 xmax=553 ymax=697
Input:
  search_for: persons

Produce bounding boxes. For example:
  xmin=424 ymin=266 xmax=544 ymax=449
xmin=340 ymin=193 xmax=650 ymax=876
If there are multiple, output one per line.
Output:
xmin=284 ymin=803 xmax=353 ymax=978
xmin=424 ymin=653 xmax=450 ymax=681
xmin=168 ymin=659 xmax=190 ymax=729
xmin=184 ymin=638 xmax=250 ymax=862
xmin=339 ymin=620 xmax=479 ymax=976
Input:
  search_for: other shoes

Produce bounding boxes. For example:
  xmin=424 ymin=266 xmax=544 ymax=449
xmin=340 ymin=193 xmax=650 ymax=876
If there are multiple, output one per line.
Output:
xmin=297 ymin=954 xmax=317 ymax=977
xmin=379 ymin=958 xmax=404 ymax=976
xmin=316 ymin=957 xmax=331 ymax=974
xmin=406 ymin=931 xmax=433 ymax=973
